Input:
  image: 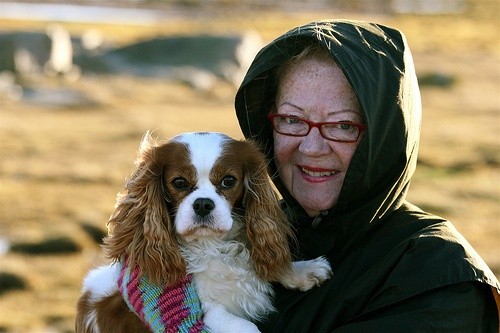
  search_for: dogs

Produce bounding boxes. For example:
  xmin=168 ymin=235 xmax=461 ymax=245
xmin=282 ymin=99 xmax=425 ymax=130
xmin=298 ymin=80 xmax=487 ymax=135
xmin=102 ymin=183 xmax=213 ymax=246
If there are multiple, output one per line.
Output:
xmin=73 ymin=127 xmax=335 ymax=333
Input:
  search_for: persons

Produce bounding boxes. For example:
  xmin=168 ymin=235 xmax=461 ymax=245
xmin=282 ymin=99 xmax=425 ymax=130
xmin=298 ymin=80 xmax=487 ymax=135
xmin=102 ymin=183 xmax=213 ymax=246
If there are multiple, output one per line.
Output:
xmin=234 ymin=20 xmax=500 ymax=333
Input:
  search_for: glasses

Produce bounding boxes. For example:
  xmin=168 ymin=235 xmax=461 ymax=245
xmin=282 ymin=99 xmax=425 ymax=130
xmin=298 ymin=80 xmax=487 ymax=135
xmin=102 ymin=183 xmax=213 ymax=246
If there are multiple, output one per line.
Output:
xmin=269 ymin=110 xmax=366 ymax=142
